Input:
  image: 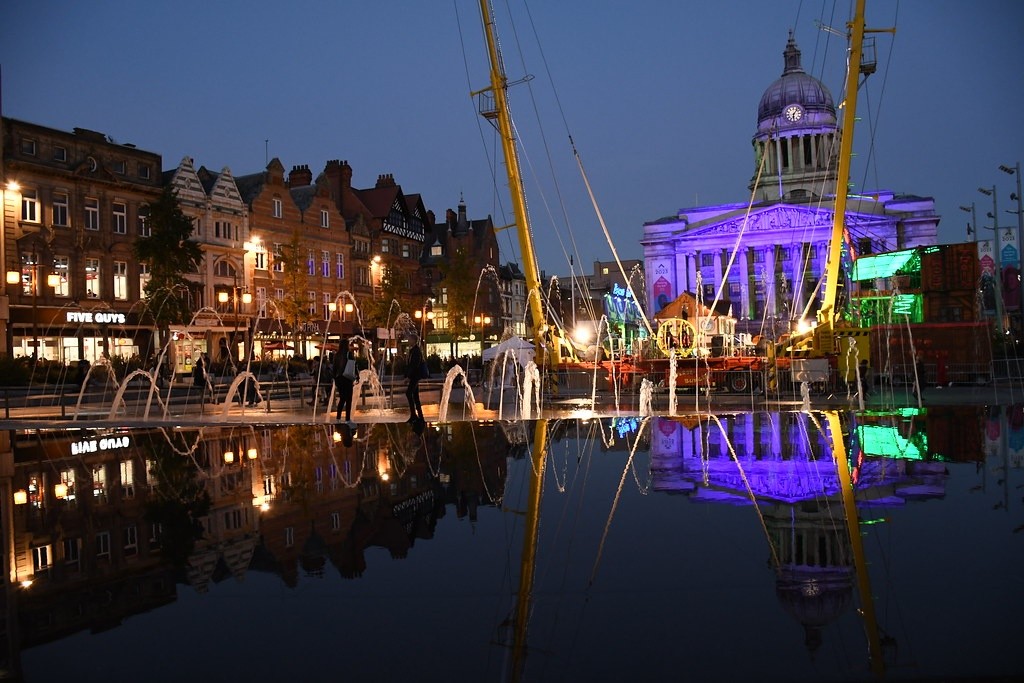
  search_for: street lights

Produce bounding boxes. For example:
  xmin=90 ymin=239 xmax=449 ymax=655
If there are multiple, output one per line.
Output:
xmin=328 ymin=293 xmax=354 ymax=343
xmin=414 ymin=305 xmax=436 ymax=360
xmin=959 ymin=162 xmax=1023 ymax=342
xmin=217 ymin=284 xmax=253 ymax=375
xmin=475 ymin=312 xmax=491 ymax=378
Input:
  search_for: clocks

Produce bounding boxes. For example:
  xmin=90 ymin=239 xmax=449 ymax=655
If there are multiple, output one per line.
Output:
xmin=800 ymin=576 xmax=823 ymax=598
xmin=782 ymin=103 xmax=805 ymax=125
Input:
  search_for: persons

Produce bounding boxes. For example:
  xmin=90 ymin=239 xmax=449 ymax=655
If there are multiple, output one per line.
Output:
xmin=934 ymin=352 xmax=953 ymax=390
xmin=332 ymin=339 xmax=360 ymax=422
xmin=909 ymin=355 xmax=927 ymax=402
xmin=403 ymin=333 xmax=429 ymax=423
xmin=193 ymin=352 xmax=211 ymax=386
xmin=280 ymin=353 xmax=336 ymax=409
xmin=854 ymin=359 xmax=869 ymax=402
xmin=237 ymin=359 xmax=258 ymax=407
xmin=483 ymin=357 xmax=524 ymax=388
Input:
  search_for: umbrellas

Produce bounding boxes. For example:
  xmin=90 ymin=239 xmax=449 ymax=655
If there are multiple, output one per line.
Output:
xmin=263 ymin=342 xmax=295 ymax=357
xmin=315 ymin=343 xmax=339 ymax=355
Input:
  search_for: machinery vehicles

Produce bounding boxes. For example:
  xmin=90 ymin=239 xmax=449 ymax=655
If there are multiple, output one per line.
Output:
xmin=452 ymin=0 xmax=989 ymax=392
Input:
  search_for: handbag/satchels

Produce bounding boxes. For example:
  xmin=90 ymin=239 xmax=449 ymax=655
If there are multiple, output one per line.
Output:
xmin=343 ymin=351 xmax=356 ymax=381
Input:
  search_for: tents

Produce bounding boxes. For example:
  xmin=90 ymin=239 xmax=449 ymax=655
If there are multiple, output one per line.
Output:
xmin=482 ymin=335 xmax=536 ymax=390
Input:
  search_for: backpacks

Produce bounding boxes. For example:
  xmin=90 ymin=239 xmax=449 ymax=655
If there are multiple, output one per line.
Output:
xmin=420 ymin=355 xmax=430 ymax=379
